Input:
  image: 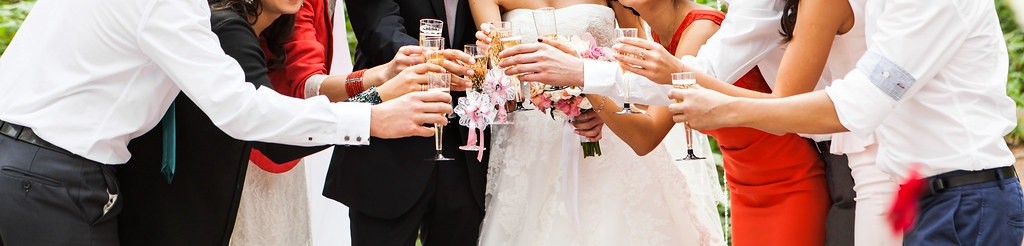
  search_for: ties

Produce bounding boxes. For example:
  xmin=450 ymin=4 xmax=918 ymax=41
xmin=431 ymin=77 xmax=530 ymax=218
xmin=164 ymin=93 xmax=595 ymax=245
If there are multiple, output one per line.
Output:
xmin=162 ymin=99 xmax=176 ymax=184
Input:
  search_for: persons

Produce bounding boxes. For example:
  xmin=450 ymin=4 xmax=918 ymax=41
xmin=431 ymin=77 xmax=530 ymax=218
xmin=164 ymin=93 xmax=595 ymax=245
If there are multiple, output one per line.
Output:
xmin=612 ymin=0 xmax=915 ymax=246
xmin=537 ymin=0 xmax=829 ymax=245
xmin=671 ymin=0 xmax=1023 ymax=246
xmin=498 ymin=0 xmax=855 ymax=245
xmin=0 ymin=0 xmax=451 ymax=246
xmin=468 ymin=0 xmax=728 ymax=246
xmin=119 ymin=1 xmax=447 ymax=246
xmin=321 ymin=0 xmax=507 ymax=246
xmin=229 ymin=0 xmax=426 ymax=244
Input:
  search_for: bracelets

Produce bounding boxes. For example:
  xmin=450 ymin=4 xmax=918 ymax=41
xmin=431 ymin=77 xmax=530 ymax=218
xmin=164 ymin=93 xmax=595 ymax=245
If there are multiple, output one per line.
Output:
xmin=345 ymin=68 xmax=368 ymax=97
xmin=347 ymin=85 xmax=382 ymax=104
xmin=592 ymin=95 xmax=607 ymax=114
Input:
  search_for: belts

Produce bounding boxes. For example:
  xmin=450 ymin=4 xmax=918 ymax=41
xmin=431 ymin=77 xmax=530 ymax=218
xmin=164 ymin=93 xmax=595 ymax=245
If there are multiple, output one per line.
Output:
xmin=0 ymin=120 xmax=71 ymax=154
xmin=918 ymin=167 xmax=1015 ymax=203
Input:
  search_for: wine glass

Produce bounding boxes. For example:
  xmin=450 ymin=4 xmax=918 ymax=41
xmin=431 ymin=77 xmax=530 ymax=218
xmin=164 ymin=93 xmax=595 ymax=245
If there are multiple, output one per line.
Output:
xmin=419 ymin=18 xmax=454 ymax=160
xmin=613 ymin=27 xmax=645 ymax=115
xmin=458 ymin=21 xmax=531 ymax=151
xmin=671 ymin=71 xmax=707 ymax=162
xmin=532 ymin=6 xmax=570 ymax=91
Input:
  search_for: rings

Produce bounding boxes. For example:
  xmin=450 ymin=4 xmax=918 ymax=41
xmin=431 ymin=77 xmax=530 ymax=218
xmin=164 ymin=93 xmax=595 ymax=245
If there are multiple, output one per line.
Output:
xmin=639 ymin=48 xmax=647 ymax=60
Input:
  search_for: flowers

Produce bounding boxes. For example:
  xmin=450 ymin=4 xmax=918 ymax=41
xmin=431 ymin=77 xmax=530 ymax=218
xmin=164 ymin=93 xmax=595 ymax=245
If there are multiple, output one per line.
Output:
xmin=529 ymin=32 xmax=615 ymax=158
